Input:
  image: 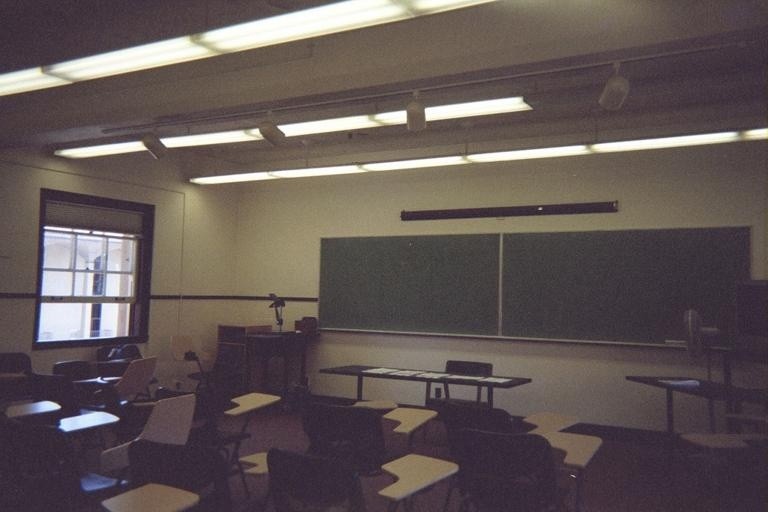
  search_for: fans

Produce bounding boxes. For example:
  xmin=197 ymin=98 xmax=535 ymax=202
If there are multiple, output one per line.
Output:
xmin=684 ymin=310 xmax=735 ymax=433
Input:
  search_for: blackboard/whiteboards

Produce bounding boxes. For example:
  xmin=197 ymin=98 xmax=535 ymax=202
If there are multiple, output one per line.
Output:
xmin=316 ymin=227 xmax=752 ymax=350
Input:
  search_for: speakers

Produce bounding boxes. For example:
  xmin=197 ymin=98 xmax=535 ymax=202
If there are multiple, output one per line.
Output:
xmin=735 ymin=280 xmax=768 ymax=337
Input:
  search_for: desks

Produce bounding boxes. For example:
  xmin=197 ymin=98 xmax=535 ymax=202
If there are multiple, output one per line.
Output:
xmin=626 ymin=376 xmax=768 ymax=487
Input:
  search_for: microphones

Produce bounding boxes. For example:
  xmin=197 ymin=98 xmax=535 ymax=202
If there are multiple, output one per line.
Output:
xmin=269 ymin=292 xmax=285 ymax=326
